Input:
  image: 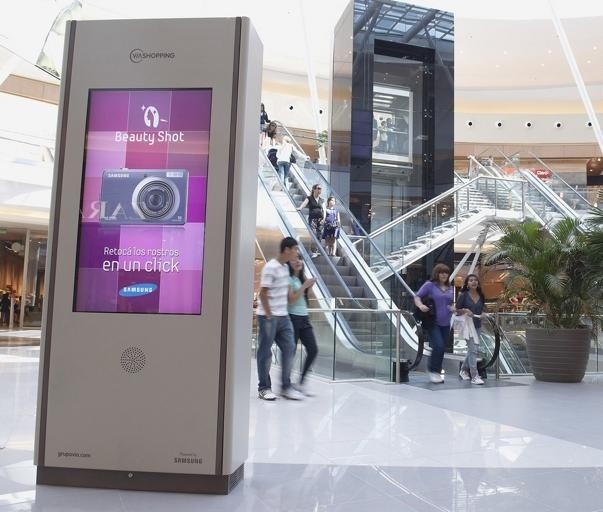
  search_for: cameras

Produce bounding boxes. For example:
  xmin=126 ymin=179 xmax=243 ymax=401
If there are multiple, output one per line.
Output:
xmin=99 ymin=169 xmax=189 ymax=226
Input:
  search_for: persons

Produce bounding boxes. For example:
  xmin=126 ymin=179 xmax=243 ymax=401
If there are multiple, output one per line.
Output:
xmin=509 ymin=291 xmax=528 ymax=312
xmin=295 ymin=184 xmax=326 ymax=258
xmin=260 ymin=103 xmax=270 ymax=131
xmin=258 ymin=121 xmax=277 ymax=155
xmin=0 ymin=285 xmax=30 ymax=326
xmin=322 ymin=197 xmax=340 ymax=256
xmin=255 ymin=237 xmax=306 ymax=400
xmin=275 ymin=135 xmax=310 ymax=191
xmin=372 ymin=114 xmax=400 ymax=154
xmin=456 ymin=274 xmax=485 ymax=384
xmin=413 ymin=264 xmax=455 ymax=383
xmin=287 ymin=253 xmax=318 ymax=395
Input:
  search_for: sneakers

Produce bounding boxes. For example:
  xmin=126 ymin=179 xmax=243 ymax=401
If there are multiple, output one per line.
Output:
xmin=429 ymin=370 xmax=484 ymax=384
xmin=259 ymin=389 xmax=276 ymax=400
xmin=281 ymin=388 xmax=303 ymax=400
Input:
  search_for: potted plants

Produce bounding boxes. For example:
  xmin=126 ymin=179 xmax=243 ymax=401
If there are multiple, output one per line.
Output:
xmin=482 ymin=208 xmax=602 ymax=383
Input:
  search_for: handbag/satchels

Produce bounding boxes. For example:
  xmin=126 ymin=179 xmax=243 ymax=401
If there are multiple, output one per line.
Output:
xmin=290 ymin=154 xmax=296 ymax=163
xmin=414 ymin=298 xmax=436 ymax=324
